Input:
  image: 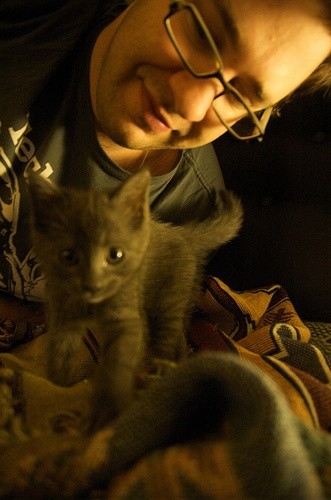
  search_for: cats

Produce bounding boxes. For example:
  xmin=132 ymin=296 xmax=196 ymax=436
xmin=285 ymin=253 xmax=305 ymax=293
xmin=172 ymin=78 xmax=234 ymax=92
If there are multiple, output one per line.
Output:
xmin=26 ymin=166 xmax=244 ymax=416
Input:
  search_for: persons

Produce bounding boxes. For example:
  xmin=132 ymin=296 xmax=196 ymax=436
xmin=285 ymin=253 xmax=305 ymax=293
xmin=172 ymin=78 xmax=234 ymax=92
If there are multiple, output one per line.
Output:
xmin=0 ymin=1 xmax=331 ymax=353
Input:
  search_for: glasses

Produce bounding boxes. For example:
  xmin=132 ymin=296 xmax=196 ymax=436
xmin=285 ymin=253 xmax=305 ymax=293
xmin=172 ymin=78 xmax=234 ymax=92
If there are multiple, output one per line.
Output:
xmin=164 ymin=0 xmax=273 ymax=141
